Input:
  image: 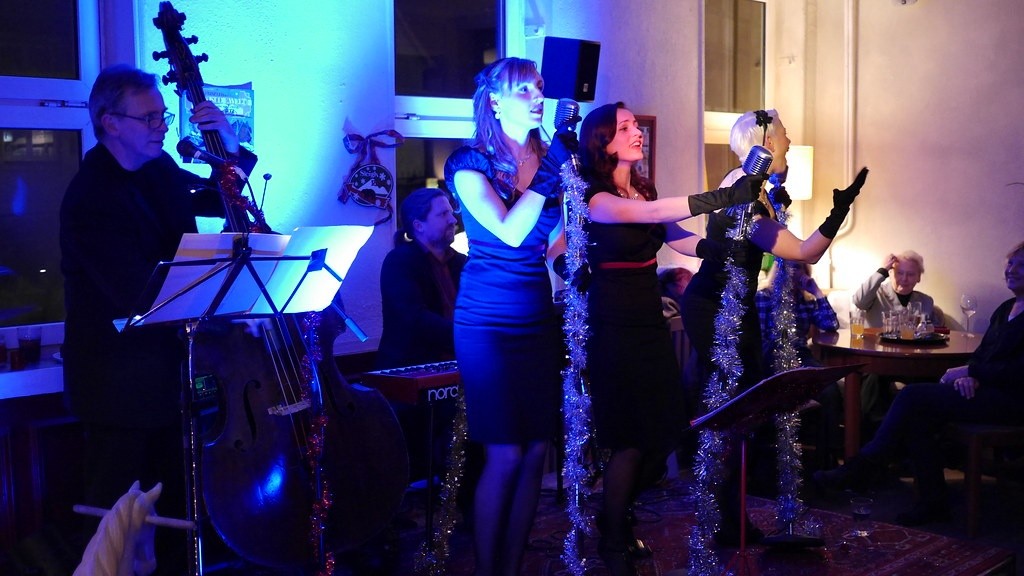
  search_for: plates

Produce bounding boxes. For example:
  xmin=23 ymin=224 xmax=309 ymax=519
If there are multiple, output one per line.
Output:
xmin=917 ymin=333 xmax=947 ymax=340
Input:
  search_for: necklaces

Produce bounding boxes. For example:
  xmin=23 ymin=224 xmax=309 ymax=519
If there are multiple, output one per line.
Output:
xmin=759 ymin=192 xmax=769 ymax=207
xmin=617 ymin=186 xmax=639 ymax=200
xmin=514 ymin=146 xmax=535 ymax=167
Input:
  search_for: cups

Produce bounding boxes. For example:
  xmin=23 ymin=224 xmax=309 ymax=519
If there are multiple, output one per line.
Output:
xmin=882 ymin=301 xmax=921 ymax=339
xmin=849 ymin=310 xmax=865 ymax=338
xmin=16 ymin=328 xmax=41 ymax=365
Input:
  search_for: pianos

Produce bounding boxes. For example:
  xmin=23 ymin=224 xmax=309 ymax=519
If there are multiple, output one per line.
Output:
xmin=361 ymin=330 xmax=579 ymax=555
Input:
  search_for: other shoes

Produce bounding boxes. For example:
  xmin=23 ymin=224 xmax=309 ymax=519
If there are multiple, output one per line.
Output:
xmin=815 ymin=467 xmax=874 ymax=496
xmin=896 ymin=498 xmax=954 ymax=525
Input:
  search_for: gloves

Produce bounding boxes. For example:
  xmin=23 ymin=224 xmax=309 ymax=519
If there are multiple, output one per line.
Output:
xmin=818 ymin=167 xmax=868 ymax=239
xmin=688 ymin=175 xmax=769 ymax=216
xmin=527 ymin=112 xmax=581 ymax=200
xmin=696 ymin=240 xmax=749 ymax=267
xmin=553 ymin=253 xmax=591 ymax=304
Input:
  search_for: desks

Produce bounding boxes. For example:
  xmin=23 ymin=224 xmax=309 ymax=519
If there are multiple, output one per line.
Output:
xmin=813 ymin=325 xmax=985 ymax=464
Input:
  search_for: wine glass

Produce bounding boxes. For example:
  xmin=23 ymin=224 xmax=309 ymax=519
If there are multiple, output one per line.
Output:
xmin=960 ymin=294 xmax=976 ymax=338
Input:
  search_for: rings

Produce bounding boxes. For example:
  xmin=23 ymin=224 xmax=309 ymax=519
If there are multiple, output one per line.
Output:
xmin=963 ymin=381 xmax=969 ymax=386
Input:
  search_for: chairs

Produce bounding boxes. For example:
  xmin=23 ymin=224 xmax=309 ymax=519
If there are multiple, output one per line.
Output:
xmin=910 ymin=422 xmax=1024 ymax=538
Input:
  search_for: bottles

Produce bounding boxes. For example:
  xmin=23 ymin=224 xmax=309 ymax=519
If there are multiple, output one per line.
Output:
xmin=0 ymin=333 xmax=7 ymax=361
xmin=917 ymin=322 xmax=926 ymax=331
xmin=925 ymin=320 xmax=934 ymax=332
xmin=915 ymin=316 xmax=920 ymax=328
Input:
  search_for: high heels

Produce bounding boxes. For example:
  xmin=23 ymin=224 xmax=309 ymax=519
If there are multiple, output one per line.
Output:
xmin=596 ymin=511 xmax=648 ymax=576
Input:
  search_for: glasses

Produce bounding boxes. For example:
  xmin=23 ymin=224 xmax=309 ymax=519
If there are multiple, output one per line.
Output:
xmin=110 ymin=111 xmax=176 ymax=128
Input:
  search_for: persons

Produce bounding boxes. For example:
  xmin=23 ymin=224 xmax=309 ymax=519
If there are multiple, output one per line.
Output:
xmin=57 ymin=66 xmax=258 ymax=560
xmin=754 ymin=261 xmax=844 ymax=469
xmin=376 ymin=187 xmax=513 ymax=510
xmin=583 ymin=100 xmax=769 ymax=576
xmin=855 ymin=251 xmax=945 ymax=419
xmin=443 ymin=57 xmax=592 ymax=576
xmin=812 ymin=243 xmax=1024 ymax=526
xmin=681 ymin=110 xmax=870 ymax=544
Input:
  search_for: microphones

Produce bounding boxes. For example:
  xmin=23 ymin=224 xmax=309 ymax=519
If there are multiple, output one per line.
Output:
xmin=741 ymin=145 xmax=773 ymax=176
xmin=176 ymin=138 xmax=248 ymax=181
xmin=554 ymin=98 xmax=580 ymax=132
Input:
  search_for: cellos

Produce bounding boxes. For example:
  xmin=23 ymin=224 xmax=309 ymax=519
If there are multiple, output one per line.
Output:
xmin=146 ymin=1 xmax=415 ymax=575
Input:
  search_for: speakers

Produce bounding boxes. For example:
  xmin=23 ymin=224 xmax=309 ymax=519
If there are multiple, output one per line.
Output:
xmin=540 ymin=36 xmax=601 ymax=103
